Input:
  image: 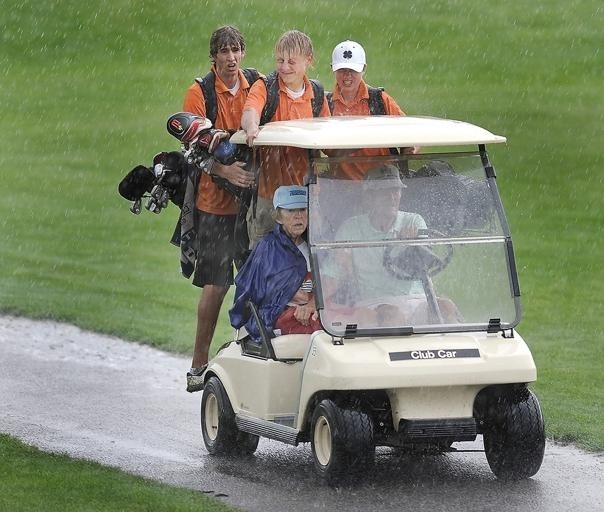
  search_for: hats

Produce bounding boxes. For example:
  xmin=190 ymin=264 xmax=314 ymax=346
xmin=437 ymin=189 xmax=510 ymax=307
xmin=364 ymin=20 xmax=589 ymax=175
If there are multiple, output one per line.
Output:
xmin=273 ymin=185 xmax=308 ymax=210
xmin=364 ymin=165 xmax=407 ymax=191
xmin=332 ymin=40 xmax=366 ymax=73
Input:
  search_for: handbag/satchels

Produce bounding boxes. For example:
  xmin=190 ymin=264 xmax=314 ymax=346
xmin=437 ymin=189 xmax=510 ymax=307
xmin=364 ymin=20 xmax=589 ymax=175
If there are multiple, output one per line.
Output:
xmin=398 ymin=160 xmax=496 ymax=237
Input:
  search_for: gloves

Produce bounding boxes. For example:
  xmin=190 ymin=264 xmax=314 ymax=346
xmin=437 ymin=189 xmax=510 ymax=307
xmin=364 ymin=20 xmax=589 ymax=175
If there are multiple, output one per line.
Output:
xmin=198 ymin=129 xmax=241 ymax=166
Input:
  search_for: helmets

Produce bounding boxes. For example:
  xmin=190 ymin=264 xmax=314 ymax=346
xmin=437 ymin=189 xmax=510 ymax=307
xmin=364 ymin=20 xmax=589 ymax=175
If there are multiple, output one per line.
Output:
xmin=167 ymin=112 xmax=213 ymax=144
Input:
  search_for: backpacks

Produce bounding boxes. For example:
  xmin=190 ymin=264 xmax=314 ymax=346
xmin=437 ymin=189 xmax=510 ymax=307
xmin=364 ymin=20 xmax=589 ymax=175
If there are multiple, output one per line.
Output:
xmin=152 ymin=67 xmax=259 ymax=209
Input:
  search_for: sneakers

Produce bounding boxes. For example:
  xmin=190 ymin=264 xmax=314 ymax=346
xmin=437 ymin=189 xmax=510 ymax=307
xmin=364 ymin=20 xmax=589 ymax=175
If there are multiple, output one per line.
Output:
xmin=186 ymin=363 xmax=210 ymax=393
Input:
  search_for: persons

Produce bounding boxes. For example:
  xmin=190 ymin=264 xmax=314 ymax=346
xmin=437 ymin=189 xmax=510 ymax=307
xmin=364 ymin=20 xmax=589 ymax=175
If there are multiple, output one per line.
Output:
xmin=240 ymin=29 xmax=364 ymax=252
xmin=331 ymin=164 xmax=465 ymax=327
xmin=322 ymin=39 xmax=418 ymax=243
xmin=229 ymin=185 xmax=381 ymax=344
xmin=182 ymin=24 xmax=267 ymax=393
